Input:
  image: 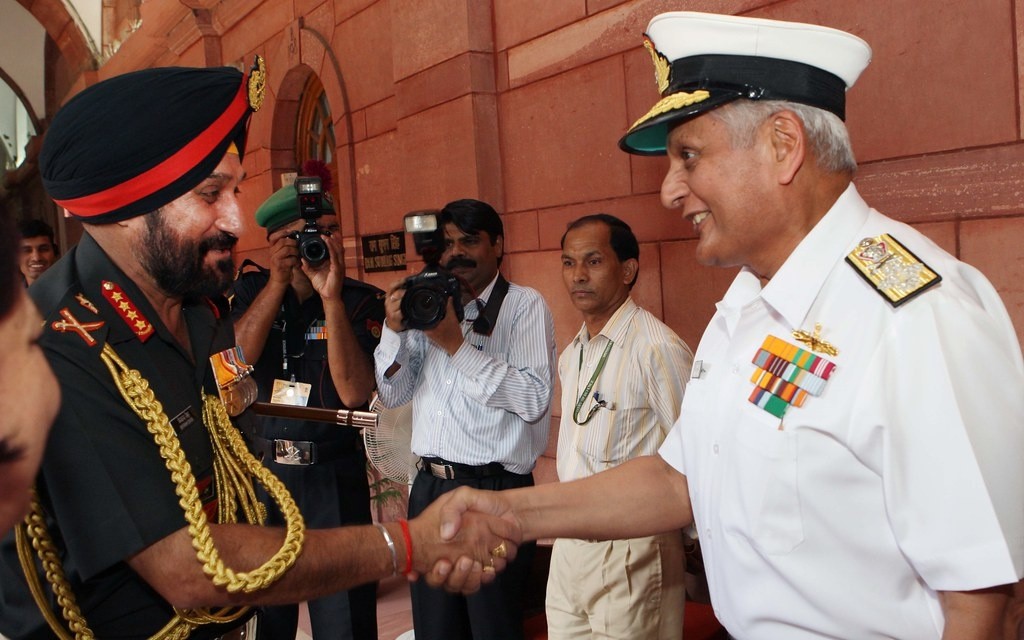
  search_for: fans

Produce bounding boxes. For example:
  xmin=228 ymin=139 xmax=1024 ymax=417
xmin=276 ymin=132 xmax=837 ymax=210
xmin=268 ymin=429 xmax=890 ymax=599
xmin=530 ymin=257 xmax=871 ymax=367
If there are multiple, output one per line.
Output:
xmin=362 ymin=394 xmax=416 ymax=640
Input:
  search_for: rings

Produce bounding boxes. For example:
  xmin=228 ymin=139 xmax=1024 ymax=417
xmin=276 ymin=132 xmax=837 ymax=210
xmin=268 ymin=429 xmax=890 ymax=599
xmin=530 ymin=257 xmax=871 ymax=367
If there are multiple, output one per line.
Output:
xmin=491 ymin=539 xmax=507 ymax=559
xmin=482 ymin=560 xmax=496 ymax=575
xmin=390 ymin=294 xmax=397 ymax=301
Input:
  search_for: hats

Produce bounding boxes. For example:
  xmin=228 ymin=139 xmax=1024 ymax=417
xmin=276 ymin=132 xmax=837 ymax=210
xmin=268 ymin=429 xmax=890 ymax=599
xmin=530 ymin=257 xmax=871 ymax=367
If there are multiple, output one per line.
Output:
xmin=617 ymin=11 xmax=873 ymax=157
xmin=38 ymin=54 xmax=267 ymax=228
xmin=254 ymin=183 xmax=336 ymax=240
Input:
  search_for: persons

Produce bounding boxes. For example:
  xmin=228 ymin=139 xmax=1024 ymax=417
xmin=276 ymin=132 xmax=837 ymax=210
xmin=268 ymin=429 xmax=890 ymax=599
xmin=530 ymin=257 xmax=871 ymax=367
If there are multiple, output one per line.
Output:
xmin=0 ymin=201 xmax=62 ymax=538
xmin=0 ymin=51 xmax=521 ymax=640
xmin=406 ymin=11 xmax=1024 ymax=640
xmin=545 ymin=214 xmax=702 ymax=640
xmin=224 ymin=159 xmax=386 ymax=640
xmin=373 ymin=199 xmax=558 ymax=640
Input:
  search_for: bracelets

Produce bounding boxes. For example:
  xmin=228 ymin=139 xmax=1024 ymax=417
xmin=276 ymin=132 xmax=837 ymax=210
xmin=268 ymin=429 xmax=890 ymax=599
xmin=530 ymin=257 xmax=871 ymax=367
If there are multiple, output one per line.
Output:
xmin=374 ymin=521 xmax=397 ymax=582
xmin=395 ymin=516 xmax=413 ymax=578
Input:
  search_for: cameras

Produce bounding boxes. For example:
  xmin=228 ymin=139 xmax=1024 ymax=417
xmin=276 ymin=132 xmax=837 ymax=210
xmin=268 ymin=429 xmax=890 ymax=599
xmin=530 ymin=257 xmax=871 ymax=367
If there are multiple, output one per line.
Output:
xmin=392 ymin=208 xmax=460 ymax=331
xmin=283 ymin=176 xmax=333 ymax=266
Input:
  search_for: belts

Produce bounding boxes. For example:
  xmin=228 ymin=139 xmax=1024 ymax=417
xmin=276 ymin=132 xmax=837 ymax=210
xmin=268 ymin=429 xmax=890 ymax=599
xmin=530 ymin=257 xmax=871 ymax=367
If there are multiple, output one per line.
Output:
xmin=420 ymin=457 xmax=504 ymax=482
xmin=254 ymin=436 xmax=356 ymax=466
